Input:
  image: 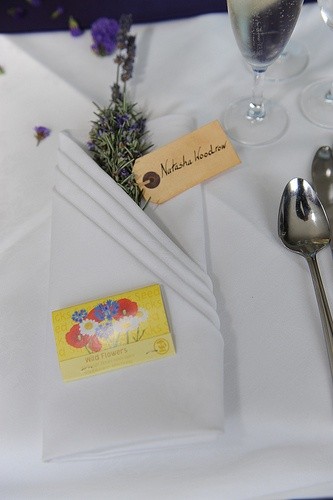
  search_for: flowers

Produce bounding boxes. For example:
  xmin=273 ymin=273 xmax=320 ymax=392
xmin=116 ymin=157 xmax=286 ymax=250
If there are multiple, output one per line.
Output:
xmin=35 ymin=8 xmax=152 ymax=215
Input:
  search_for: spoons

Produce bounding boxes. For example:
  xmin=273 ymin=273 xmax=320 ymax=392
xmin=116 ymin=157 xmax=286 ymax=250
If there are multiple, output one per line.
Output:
xmin=278 ymin=146 xmax=333 ymax=380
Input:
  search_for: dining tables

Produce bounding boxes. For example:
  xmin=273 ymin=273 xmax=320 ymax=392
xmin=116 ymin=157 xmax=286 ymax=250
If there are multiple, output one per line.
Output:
xmin=0 ymin=0 xmax=333 ymax=500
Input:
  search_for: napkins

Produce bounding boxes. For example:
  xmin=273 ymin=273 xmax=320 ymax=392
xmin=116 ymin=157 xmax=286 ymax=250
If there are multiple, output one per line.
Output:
xmin=40 ymin=109 xmax=225 ymax=464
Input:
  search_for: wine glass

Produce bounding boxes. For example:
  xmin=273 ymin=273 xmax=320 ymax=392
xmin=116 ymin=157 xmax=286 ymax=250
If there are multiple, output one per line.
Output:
xmin=223 ymin=0 xmax=304 ymax=146
xmin=299 ymin=0 xmax=333 ymax=129
